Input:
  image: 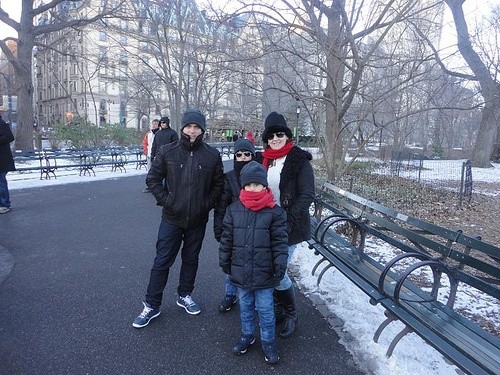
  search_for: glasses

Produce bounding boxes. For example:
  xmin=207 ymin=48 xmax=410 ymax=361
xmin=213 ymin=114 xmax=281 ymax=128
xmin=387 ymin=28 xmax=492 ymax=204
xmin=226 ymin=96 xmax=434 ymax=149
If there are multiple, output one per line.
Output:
xmin=267 ymin=132 xmax=286 ymax=140
xmin=160 ymin=122 xmax=166 ymax=124
xmin=234 ymin=152 xmax=252 ymax=158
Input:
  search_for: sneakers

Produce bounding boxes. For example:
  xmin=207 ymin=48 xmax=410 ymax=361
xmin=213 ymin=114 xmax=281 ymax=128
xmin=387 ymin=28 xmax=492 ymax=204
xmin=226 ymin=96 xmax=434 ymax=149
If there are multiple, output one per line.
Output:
xmin=218 ymin=294 xmax=237 ymax=313
xmin=262 ymin=344 xmax=279 ymax=364
xmin=176 ymin=295 xmax=201 ymax=315
xmin=133 ymin=301 xmax=161 ymax=329
xmin=232 ymin=334 xmax=255 ymax=353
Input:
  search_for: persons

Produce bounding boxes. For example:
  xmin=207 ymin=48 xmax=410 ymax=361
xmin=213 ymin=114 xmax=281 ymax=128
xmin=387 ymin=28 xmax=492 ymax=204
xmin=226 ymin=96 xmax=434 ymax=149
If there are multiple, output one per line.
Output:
xmin=214 ymin=138 xmax=263 ymax=313
xmin=219 ymin=131 xmax=238 ymax=142
xmin=219 ymin=161 xmax=289 ymax=364
xmin=143 ymin=118 xmax=160 ymax=193
xmin=132 ymin=108 xmax=224 ymax=328
xmin=261 ymin=111 xmax=316 ymax=339
xmin=0 ymin=113 xmax=16 ymax=213
xmin=151 ymin=116 xmax=179 ymax=206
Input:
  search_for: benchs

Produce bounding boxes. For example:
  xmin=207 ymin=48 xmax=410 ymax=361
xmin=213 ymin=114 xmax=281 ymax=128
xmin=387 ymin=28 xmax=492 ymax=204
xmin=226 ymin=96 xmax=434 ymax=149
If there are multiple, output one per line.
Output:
xmin=307 ymin=180 xmax=500 ymax=375
xmin=13 ymin=147 xmax=146 ymax=180
xmin=208 ymin=141 xmax=236 ymax=157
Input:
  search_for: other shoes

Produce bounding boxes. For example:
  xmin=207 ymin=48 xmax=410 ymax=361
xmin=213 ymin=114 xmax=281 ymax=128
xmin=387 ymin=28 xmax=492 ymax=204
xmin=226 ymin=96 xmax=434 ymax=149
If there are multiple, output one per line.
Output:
xmin=143 ymin=187 xmax=150 ymax=192
xmin=0 ymin=207 xmax=11 ymax=213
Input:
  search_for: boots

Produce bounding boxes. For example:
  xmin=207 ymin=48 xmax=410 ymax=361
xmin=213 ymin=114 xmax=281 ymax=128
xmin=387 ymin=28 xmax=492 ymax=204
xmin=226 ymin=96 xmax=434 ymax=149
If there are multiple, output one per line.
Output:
xmin=273 ymin=289 xmax=283 ymax=326
xmin=280 ymin=284 xmax=298 ymax=338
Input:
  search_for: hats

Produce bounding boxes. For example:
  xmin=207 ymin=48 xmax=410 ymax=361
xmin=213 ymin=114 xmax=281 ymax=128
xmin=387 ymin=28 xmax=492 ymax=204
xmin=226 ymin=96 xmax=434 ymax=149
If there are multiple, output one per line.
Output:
xmin=265 ymin=112 xmax=288 ymax=129
xmin=233 ymin=139 xmax=256 ymax=156
xmin=239 ymin=160 xmax=268 ymax=188
xmin=159 ymin=117 xmax=169 ymax=124
xmin=181 ymin=109 xmax=206 ymax=132
